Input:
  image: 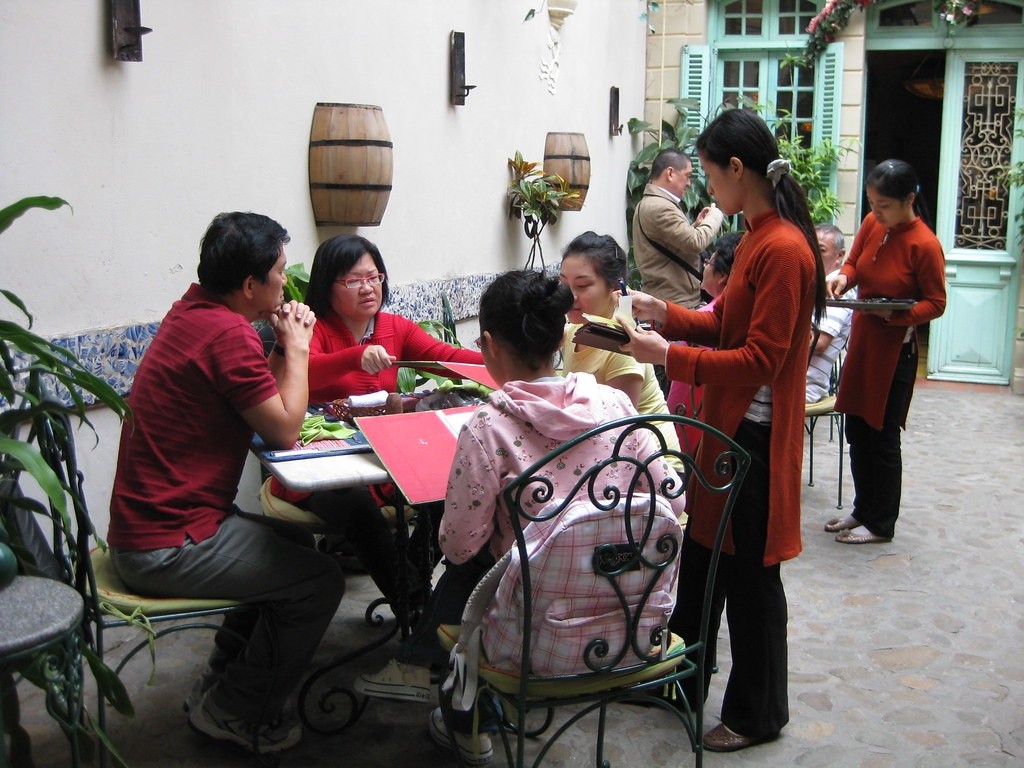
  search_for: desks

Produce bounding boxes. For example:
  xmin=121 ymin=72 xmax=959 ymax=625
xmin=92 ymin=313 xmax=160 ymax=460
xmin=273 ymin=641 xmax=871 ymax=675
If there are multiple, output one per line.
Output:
xmin=246 ymin=400 xmax=555 ymax=739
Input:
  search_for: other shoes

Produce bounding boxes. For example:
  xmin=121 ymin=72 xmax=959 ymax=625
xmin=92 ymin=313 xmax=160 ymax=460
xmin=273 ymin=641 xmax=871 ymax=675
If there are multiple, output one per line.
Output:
xmin=701 ymin=725 xmax=779 ymax=753
xmin=835 ymin=529 xmax=892 ymax=544
xmin=824 ymin=516 xmax=859 ymax=533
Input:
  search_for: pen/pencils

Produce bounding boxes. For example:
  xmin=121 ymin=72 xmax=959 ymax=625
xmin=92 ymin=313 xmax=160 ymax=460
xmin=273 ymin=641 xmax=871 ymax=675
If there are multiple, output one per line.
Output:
xmin=618 ymin=277 xmax=639 ymax=325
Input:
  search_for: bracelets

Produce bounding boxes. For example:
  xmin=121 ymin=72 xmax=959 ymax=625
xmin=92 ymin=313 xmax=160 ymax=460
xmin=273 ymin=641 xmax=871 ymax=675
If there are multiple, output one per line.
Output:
xmin=272 ymin=343 xmax=285 ymax=357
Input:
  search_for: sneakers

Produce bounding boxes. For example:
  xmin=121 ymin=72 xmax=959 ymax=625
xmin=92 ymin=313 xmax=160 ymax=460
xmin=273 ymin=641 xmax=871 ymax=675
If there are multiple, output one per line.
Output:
xmin=429 ymin=706 xmax=494 ymax=764
xmin=183 ymin=672 xmax=218 ymax=713
xmin=355 ymin=658 xmax=432 ymax=702
xmin=188 ymin=681 xmax=302 ymax=754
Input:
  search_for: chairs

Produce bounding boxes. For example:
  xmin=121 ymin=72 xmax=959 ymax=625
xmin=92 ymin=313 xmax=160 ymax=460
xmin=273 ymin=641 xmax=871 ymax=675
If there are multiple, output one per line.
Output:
xmin=805 ymin=335 xmax=851 ymax=511
xmin=31 ymin=396 xmax=295 ymax=768
xmin=259 ymin=462 xmax=419 ymax=549
xmin=436 ymin=415 xmax=751 ymax=768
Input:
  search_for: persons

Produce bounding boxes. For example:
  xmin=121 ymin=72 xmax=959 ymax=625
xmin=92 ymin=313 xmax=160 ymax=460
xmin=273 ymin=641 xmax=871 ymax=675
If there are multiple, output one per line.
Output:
xmin=476 ymin=230 xmax=689 ymax=494
xmin=823 ymin=158 xmax=947 ymax=544
xmin=108 ymin=212 xmax=347 ymax=753
xmin=610 ymin=108 xmax=828 ymax=753
xmin=805 ymin=223 xmax=857 ymax=404
xmin=267 ymin=234 xmax=488 ymax=705
xmin=633 ymin=147 xmax=724 ymax=401
xmin=353 ymin=270 xmax=688 ymax=765
xmin=667 ymin=231 xmax=747 ymax=454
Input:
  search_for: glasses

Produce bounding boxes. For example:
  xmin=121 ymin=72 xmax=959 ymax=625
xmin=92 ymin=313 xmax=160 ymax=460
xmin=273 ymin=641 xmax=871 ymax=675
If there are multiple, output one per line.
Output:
xmin=336 ymin=274 xmax=384 ymax=289
xmin=703 ymin=259 xmax=717 ymax=269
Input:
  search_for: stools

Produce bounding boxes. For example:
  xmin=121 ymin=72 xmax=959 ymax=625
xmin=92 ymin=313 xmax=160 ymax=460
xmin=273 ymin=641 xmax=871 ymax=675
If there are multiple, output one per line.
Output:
xmin=0 ymin=575 xmax=85 ymax=768
